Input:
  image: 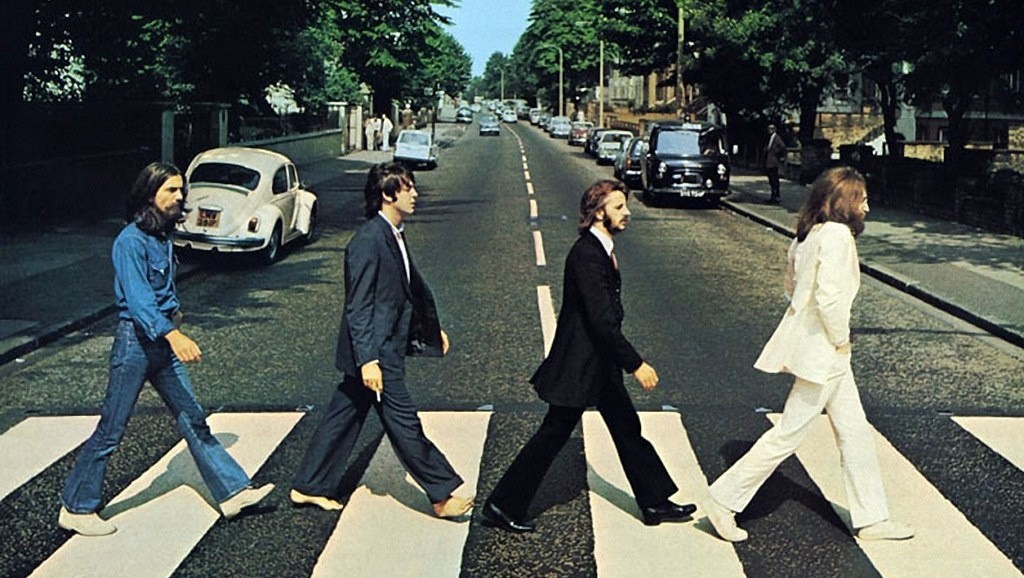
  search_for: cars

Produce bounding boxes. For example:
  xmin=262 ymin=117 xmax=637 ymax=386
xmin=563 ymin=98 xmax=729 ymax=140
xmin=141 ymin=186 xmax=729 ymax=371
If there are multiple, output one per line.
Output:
xmin=472 ymin=99 xmax=532 ymax=124
xmin=457 ymin=106 xmax=475 ymax=125
xmin=584 ymin=126 xmax=644 ymax=184
xmin=169 ymin=147 xmax=319 ymax=263
xmin=528 ymin=108 xmax=572 ymax=140
xmin=641 ymin=121 xmax=739 ymax=206
xmin=567 ymin=122 xmax=594 ymax=146
xmin=392 ymin=130 xmax=440 ymax=169
xmin=478 ymin=115 xmax=500 ymax=137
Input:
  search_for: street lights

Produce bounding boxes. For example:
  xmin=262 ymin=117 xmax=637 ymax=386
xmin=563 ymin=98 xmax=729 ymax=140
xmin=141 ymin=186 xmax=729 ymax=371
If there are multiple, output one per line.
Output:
xmin=543 ymin=44 xmax=563 ymax=117
xmin=576 ymin=20 xmax=604 ymax=127
xmin=494 ymin=67 xmax=504 ymax=101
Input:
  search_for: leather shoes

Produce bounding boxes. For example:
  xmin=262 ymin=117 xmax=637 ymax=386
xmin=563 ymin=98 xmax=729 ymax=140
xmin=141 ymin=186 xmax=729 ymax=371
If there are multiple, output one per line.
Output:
xmin=482 ymin=499 xmax=537 ymax=532
xmin=642 ymin=500 xmax=697 ymax=526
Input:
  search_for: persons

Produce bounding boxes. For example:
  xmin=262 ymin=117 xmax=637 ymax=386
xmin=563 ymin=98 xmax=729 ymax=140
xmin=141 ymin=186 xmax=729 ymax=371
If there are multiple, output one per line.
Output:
xmin=59 ymin=162 xmax=276 ymax=535
xmin=482 ymin=181 xmax=699 ymax=534
xmin=701 ymin=167 xmax=914 ymax=541
xmin=289 ymin=161 xmax=474 ymax=518
xmin=765 ymin=125 xmax=787 ymax=205
xmin=362 ymin=104 xmax=418 ymax=152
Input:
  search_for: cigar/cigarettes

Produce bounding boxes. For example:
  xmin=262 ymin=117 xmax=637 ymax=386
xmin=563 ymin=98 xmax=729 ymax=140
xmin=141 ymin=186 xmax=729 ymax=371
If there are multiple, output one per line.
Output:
xmin=376 ymin=387 xmax=380 ymax=402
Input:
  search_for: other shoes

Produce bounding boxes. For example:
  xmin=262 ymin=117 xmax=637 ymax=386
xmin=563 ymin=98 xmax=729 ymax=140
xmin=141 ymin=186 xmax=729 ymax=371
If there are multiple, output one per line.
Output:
xmin=219 ymin=483 xmax=276 ymax=519
xmin=59 ymin=507 xmax=118 ymax=536
xmin=858 ymin=518 xmax=914 ymax=540
xmin=702 ymin=493 xmax=749 ymax=542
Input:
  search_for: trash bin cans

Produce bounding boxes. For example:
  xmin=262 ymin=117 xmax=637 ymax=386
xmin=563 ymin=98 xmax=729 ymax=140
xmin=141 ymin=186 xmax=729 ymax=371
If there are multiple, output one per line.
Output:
xmin=799 ymin=139 xmax=833 ymax=186
xmin=838 ymin=144 xmax=875 ymax=168
